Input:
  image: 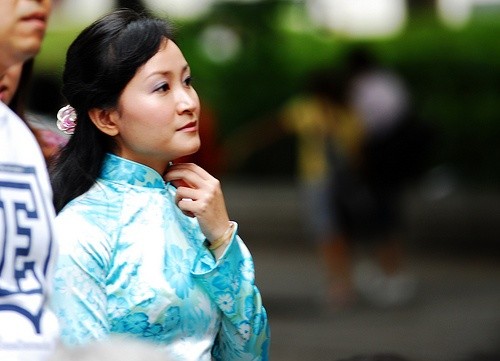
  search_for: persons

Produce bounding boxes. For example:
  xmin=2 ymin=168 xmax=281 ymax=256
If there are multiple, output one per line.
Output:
xmin=0 ymin=57 xmax=52 ymax=157
xmin=337 ymin=40 xmax=413 ymax=308
xmin=50 ymin=7 xmax=270 ymax=361
xmin=279 ymin=65 xmax=367 ymax=308
xmin=0 ymin=0 xmax=60 ymax=361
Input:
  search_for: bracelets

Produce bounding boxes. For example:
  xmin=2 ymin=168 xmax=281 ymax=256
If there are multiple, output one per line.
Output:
xmin=207 ymin=222 xmax=233 ymax=251
